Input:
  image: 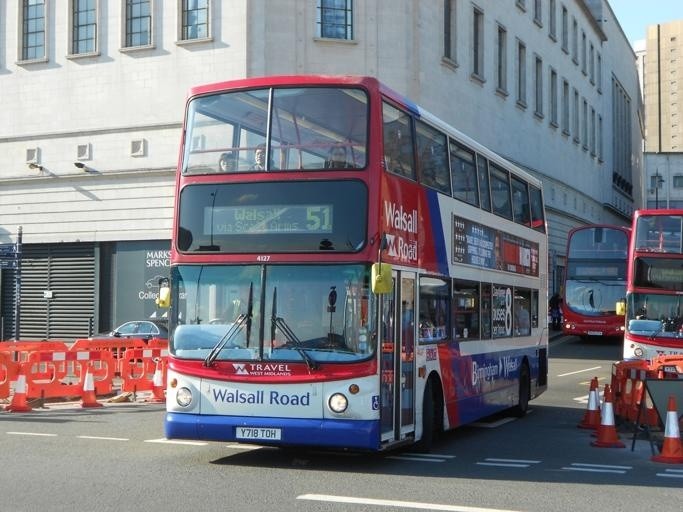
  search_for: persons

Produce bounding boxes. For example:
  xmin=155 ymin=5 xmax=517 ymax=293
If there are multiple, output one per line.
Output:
xmin=636 ymin=300 xmax=659 ymax=320
xmin=216 ymin=153 xmax=237 ymax=173
xmin=248 ymin=144 xmax=279 ymax=171
xmin=549 ymin=294 xmax=563 ymax=330
xmin=223 ymin=281 xmax=261 ymax=329
xmin=327 ymin=142 xmax=353 ymax=169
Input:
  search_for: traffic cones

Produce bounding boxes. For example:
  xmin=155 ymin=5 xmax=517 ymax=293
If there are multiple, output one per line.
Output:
xmin=575 ymin=379 xmax=603 ymax=431
xmin=4 ymin=362 xmax=39 ymax=412
xmin=647 ymin=394 xmax=682 ymax=465
xmin=590 ymin=382 xmax=621 ymax=437
xmin=589 ymin=390 xmax=627 ymax=448
xmin=142 ymin=358 xmax=166 ymax=403
xmin=581 ymin=375 xmax=601 ymax=417
xmin=77 ymin=362 xmax=105 ymax=408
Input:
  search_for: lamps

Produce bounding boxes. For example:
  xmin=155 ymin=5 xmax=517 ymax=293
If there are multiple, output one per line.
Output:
xmin=74 ymin=162 xmax=84 ymax=169
xmin=29 ymin=164 xmax=42 ymax=172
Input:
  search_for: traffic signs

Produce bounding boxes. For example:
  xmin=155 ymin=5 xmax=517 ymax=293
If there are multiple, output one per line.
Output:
xmin=0 ymin=260 xmax=19 ymax=270
xmin=0 ymin=242 xmax=16 ymax=259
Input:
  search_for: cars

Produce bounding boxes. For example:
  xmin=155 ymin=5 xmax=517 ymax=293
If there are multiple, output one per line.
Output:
xmin=85 ymin=318 xmax=185 ymax=340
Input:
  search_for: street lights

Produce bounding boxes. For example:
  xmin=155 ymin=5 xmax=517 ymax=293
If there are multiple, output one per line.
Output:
xmin=655 ymin=167 xmax=665 ymax=211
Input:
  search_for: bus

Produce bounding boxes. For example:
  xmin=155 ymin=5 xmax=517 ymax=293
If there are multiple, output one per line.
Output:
xmin=160 ymin=70 xmax=551 ymax=461
xmin=619 ymin=207 xmax=682 ymax=377
xmin=558 ymin=222 xmax=630 ymax=340
